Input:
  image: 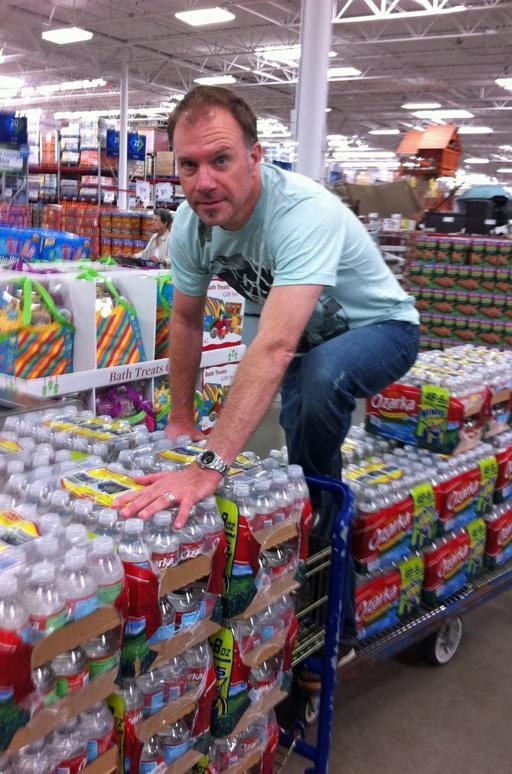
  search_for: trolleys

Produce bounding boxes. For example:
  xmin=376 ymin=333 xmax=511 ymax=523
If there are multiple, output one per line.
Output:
xmin=294 ymin=558 xmax=512 ymax=729
xmin=271 ymin=473 xmax=353 ymax=773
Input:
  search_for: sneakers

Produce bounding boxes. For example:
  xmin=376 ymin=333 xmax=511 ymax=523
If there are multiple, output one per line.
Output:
xmin=300 ymin=644 xmax=355 ymax=689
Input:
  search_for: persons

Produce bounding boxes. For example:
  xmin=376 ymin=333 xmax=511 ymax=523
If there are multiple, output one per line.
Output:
xmin=107 ymin=87 xmax=422 ymax=687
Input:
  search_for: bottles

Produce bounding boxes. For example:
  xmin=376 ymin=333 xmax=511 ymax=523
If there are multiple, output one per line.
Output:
xmin=1 ymin=403 xmax=308 ymax=774
xmin=340 ymin=342 xmax=511 ymax=638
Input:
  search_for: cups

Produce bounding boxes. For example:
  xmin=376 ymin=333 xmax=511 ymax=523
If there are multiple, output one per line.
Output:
xmin=390 ymin=213 xmax=402 ymax=231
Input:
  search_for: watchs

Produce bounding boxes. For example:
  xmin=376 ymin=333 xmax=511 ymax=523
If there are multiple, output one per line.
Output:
xmin=196 ymin=449 xmax=231 ymax=475
xmin=119 ymin=206 xmax=172 ymax=267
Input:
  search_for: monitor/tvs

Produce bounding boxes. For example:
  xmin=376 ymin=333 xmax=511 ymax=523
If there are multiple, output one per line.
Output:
xmin=424 ymin=212 xmax=467 ymax=234
